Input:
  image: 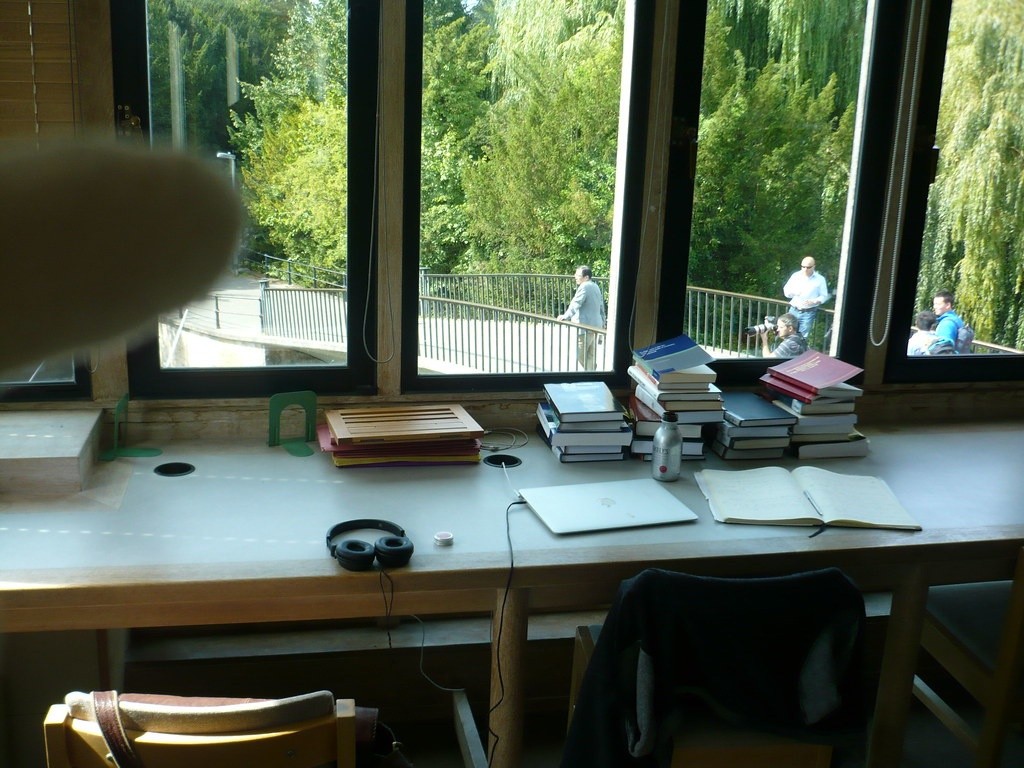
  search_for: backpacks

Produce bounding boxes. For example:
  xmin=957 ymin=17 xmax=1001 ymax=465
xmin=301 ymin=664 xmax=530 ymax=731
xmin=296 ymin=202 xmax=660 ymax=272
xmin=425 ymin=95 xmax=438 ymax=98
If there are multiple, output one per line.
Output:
xmin=946 ymin=319 xmax=974 ymax=354
xmin=912 ymin=334 xmax=958 ymax=355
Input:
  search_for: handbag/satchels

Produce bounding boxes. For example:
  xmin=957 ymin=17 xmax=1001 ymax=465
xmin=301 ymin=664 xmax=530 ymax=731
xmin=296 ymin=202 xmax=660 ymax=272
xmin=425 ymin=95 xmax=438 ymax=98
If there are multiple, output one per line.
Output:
xmin=117 ymin=693 xmax=379 ymax=768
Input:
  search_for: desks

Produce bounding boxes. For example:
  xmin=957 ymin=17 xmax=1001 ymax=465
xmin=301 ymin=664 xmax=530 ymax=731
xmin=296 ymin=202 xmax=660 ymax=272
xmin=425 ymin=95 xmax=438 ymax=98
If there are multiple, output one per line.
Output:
xmin=0 ymin=415 xmax=1024 ymax=768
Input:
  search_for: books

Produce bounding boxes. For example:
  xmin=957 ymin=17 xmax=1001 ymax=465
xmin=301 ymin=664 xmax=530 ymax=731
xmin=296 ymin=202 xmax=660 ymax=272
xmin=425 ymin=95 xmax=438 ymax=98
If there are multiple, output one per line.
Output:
xmin=705 ymin=390 xmax=799 ymax=461
xmin=761 ymin=349 xmax=871 ymax=458
xmin=629 ymin=332 xmax=727 ymax=461
xmin=535 ymin=381 xmax=634 ymax=463
xmin=695 ymin=465 xmax=923 ymax=533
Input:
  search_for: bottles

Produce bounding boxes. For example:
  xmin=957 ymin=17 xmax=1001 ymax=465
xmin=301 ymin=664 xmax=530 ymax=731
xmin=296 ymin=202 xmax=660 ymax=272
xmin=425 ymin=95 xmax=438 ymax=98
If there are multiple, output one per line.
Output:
xmin=652 ymin=411 xmax=682 ymax=482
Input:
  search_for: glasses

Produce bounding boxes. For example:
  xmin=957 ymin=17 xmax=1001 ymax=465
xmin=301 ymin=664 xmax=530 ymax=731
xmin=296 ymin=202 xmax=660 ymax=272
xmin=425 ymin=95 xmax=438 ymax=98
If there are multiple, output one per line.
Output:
xmin=801 ymin=266 xmax=814 ymax=269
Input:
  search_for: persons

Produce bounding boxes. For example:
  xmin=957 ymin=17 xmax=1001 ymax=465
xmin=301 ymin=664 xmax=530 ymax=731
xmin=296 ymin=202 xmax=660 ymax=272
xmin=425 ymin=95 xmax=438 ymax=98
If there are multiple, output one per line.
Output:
xmin=782 ymin=257 xmax=830 ymax=344
xmin=760 ymin=312 xmax=809 ymax=359
xmin=907 ymin=293 xmax=974 ymax=356
xmin=556 ymin=264 xmax=605 ymax=371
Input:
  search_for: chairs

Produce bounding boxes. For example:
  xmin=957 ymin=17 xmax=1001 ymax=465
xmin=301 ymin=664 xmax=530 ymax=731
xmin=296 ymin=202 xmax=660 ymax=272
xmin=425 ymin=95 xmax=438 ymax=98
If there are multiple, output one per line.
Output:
xmin=557 ymin=570 xmax=871 ymax=768
xmin=43 ymin=688 xmax=358 ymax=768
xmin=908 ymin=548 xmax=1024 ymax=768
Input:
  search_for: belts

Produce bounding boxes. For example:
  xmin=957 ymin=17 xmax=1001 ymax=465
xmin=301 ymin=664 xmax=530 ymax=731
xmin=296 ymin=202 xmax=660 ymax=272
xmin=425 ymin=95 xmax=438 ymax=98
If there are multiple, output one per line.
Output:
xmin=792 ymin=306 xmax=815 ymax=313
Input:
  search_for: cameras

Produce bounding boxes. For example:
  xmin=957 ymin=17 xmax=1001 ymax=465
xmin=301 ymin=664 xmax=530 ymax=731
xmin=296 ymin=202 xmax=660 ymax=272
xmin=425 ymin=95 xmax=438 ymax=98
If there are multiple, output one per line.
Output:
xmin=744 ymin=316 xmax=777 ymax=337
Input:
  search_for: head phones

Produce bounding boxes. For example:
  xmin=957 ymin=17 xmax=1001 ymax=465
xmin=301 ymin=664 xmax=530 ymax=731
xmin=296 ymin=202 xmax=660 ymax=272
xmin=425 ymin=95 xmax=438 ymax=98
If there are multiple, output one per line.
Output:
xmin=326 ymin=518 xmax=413 ymax=572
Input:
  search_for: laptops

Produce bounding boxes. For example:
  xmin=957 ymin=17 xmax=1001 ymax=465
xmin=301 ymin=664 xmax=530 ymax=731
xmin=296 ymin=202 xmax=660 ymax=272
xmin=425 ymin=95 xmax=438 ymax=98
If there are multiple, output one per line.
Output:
xmin=519 ymin=478 xmax=699 ymax=534
